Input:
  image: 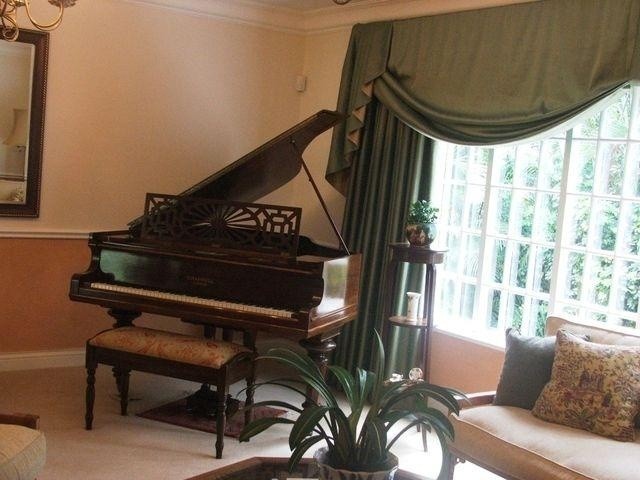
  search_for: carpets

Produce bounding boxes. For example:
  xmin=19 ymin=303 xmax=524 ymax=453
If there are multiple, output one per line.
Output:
xmin=135 ymin=390 xmax=290 ymax=442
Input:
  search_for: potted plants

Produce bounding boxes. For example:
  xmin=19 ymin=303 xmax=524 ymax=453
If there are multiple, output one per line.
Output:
xmin=402 ymin=197 xmax=442 ymax=247
xmin=230 ymin=338 xmax=475 ymax=480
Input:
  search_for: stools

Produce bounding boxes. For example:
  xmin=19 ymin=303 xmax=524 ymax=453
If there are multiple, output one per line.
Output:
xmin=83 ymin=323 xmax=260 ymax=461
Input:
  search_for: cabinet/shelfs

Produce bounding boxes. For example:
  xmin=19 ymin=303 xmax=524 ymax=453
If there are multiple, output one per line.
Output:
xmin=381 ymin=242 xmax=446 ymax=412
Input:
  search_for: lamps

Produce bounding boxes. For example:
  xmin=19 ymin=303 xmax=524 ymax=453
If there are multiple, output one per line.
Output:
xmin=0 ymin=0 xmax=78 ymax=42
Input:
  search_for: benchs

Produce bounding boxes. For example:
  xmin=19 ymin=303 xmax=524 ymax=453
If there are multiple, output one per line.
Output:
xmin=438 ymin=313 xmax=640 ymax=480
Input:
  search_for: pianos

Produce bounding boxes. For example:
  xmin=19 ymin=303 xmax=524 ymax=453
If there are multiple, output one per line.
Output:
xmin=68 ymin=110 xmax=368 ymax=435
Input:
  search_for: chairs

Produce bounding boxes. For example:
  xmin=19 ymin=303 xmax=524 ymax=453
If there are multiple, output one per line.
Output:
xmin=0 ymin=409 xmax=47 ymax=480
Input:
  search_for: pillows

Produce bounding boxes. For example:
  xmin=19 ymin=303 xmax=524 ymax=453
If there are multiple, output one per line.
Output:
xmin=530 ymin=329 xmax=640 ymax=443
xmin=490 ymin=327 xmax=593 ymax=411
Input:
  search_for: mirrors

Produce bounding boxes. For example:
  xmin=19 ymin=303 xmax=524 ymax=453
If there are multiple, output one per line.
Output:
xmin=0 ymin=21 xmax=52 ymax=220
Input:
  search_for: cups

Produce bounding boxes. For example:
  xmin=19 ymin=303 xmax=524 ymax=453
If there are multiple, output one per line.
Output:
xmin=406 ymin=292 xmax=422 ymax=323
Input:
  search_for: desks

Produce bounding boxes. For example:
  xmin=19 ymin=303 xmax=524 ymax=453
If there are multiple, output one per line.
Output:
xmin=183 ymin=456 xmax=435 ymax=480
